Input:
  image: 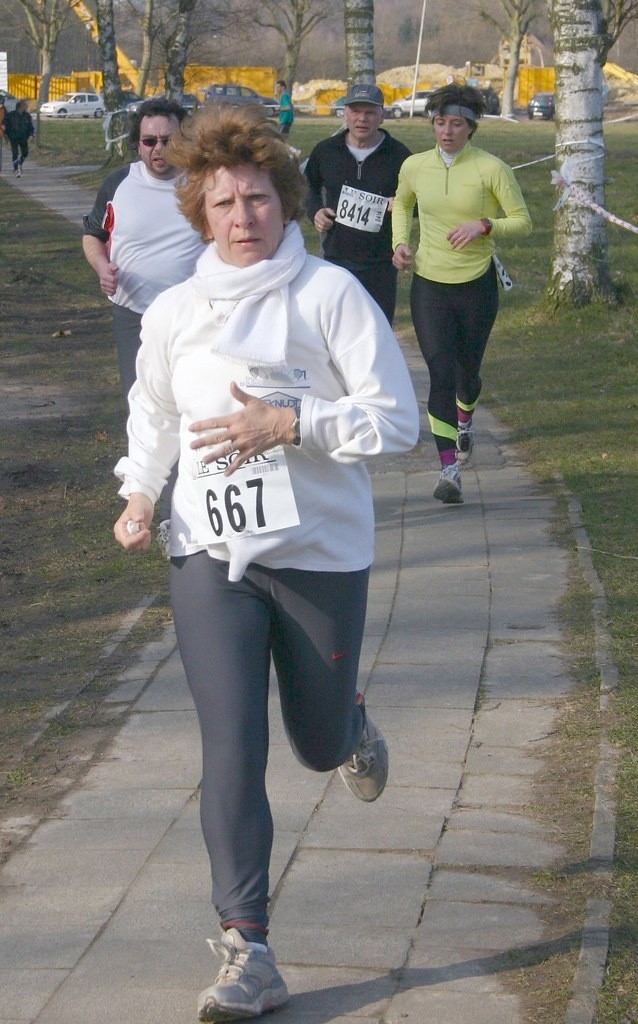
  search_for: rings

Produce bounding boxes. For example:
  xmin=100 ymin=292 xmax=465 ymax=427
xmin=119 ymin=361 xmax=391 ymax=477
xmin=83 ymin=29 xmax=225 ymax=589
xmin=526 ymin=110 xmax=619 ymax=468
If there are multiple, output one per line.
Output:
xmin=318 ymin=225 xmax=321 ymax=229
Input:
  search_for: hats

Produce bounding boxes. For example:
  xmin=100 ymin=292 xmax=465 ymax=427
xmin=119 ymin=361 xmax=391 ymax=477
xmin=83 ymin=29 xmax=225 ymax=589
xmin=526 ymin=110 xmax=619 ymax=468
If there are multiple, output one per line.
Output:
xmin=343 ymin=83 xmax=385 ymax=107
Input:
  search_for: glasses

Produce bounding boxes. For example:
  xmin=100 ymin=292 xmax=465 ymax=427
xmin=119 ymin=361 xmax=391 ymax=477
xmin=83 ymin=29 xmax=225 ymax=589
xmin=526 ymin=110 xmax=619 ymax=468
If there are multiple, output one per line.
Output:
xmin=138 ymin=137 xmax=170 ymax=148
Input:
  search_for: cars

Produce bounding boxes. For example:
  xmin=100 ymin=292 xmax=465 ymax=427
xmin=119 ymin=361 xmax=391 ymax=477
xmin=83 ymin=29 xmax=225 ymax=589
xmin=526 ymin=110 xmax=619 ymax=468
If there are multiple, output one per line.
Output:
xmin=390 ymin=89 xmax=437 ymax=119
xmin=126 ymin=93 xmax=201 ymax=115
xmin=334 ymin=95 xmax=392 ymax=119
xmin=477 ymin=88 xmax=499 ymax=115
xmin=39 ymin=92 xmax=107 ymax=119
xmin=0 ymin=88 xmax=21 ymax=120
xmin=526 ymin=91 xmax=555 ymax=121
xmin=121 ymin=90 xmax=142 ymax=110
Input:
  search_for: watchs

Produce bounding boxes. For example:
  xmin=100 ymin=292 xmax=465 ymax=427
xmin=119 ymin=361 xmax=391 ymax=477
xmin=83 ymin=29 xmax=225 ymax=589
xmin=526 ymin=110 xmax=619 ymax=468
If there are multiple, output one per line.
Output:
xmin=481 ymin=218 xmax=492 ymax=236
xmin=290 ymin=407 xmax=301 ymax=445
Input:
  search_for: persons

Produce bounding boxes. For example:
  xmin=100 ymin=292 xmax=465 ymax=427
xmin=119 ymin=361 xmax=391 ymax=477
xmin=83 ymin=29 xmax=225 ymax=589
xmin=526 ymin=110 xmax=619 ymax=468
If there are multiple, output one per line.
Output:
xmin=0 ymin=95 xmax=34 ymax=178
xmin=80 ymin=80 xmax=531 ymax=1024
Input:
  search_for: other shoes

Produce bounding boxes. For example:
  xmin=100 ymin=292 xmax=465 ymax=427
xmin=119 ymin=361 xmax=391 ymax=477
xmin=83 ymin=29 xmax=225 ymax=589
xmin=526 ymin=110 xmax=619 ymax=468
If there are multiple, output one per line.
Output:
xmin=14 ymin=170 xmax=22 ymax=178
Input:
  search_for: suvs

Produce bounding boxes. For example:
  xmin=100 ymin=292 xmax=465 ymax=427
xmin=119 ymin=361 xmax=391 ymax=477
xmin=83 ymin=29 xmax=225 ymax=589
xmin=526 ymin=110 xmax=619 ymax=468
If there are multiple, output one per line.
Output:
xmin=204 ymin=83 xmax=280 ymax=118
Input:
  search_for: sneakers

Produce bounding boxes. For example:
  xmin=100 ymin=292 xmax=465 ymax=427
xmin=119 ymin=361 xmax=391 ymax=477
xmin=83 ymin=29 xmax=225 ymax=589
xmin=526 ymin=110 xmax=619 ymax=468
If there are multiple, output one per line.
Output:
xmin=196 ymin=926 xmax=290 ymax=1024
xmin=431 ymin=462 xmax=465 ymax=504
xmin=454 ymin=416 xmax=474 ymax=465
xmin=336 ymin=686 xmax=390 ymax=802
